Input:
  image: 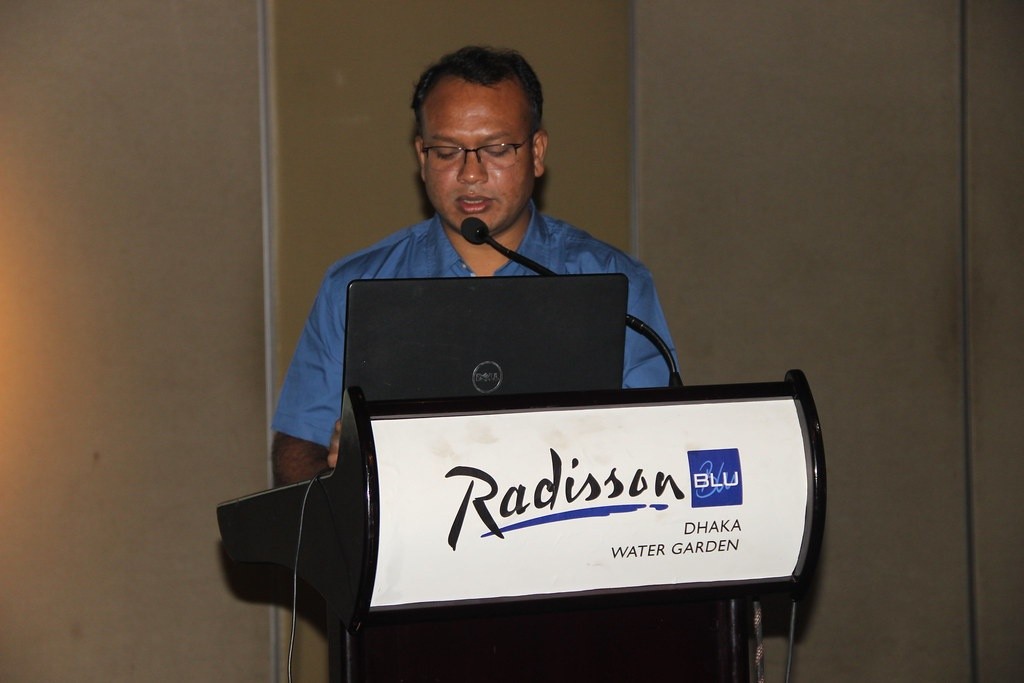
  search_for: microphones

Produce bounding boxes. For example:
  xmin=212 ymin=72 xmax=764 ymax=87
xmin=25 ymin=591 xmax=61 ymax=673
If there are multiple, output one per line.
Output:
xmin=461 ymin=217 xmax=682 ymax=387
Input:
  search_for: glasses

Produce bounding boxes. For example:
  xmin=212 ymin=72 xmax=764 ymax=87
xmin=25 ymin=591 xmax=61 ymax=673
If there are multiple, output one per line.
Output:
xmin=421 ymin=134 xmax=533 ymax=170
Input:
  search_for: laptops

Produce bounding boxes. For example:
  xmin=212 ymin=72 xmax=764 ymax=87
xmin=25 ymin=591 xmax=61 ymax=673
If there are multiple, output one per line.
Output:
xmin=342 ymin=272 xmax=629 ymax=403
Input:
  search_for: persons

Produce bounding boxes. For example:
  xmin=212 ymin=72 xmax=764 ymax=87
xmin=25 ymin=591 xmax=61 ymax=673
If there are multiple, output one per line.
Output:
xmin=271 ymin=43 xmax=681 ymax=489
